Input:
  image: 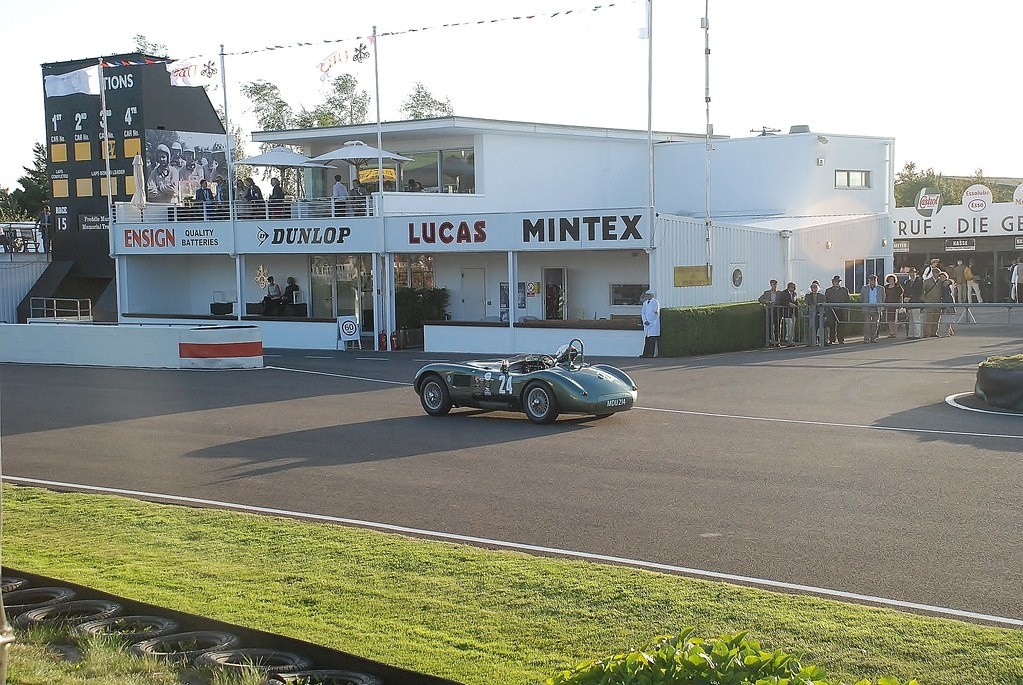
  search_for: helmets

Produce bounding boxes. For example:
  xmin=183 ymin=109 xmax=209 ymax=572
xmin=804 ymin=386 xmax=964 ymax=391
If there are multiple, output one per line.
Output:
xmin=183 ymin=144 xmax=195 ymax=159
xmin=156 ymin=144 xmax=170 ymax=162
xmin=202 ymin=146 xmax=212 ymax=153
xmin=195 ymin=145 xmax=202 ymax=152
xmin=210 ymin=142 xmax=226 ymax=154
xmin=171 ymin=142 xmax=182 ymax=155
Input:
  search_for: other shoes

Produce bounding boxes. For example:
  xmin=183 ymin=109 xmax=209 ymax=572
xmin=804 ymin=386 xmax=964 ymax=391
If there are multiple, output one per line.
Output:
xmin=871 ymin=338 xmax=877 ymax=342
xmin=639 ymin=353 xmax=653 ymax=358
xmin=864 ymin=339 xmax=869 ymax=343
xmin=838 ymin=340 xmax=844 ymax=344
xmin=887 ymin=334 xmax=892 ymax=338
xmin=893 ymin=335 xmax=896 ymax=337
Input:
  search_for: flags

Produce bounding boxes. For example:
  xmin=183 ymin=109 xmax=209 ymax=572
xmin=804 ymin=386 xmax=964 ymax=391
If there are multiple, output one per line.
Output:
xmin=166 ymin=52 xmax=222 ymax=87
xmin=44 ymin=65 xmax=99 ymax=99
xmin=316 ymin=35 xmax=376 ymax=82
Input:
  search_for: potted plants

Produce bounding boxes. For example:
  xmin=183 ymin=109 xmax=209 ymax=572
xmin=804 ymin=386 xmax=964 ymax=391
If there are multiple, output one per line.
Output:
xmin=394 ymin=286 xmax=449 ymax=349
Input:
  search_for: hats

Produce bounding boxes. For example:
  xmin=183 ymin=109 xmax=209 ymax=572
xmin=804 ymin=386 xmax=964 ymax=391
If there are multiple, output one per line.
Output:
xmin=931 ymin=258 xmax=939 ymax=264
xmin=646 ymin=290 xmax=654 ymax=294
xmin=940 ymin=272 xmax=949 ymax=280
xmin=907 ymin=267 xmax=919 ymax=273
xmin=832 ymin=276 xmax=842 ymax=281
xmin=868 ymin=275 xmax=878 ymax=279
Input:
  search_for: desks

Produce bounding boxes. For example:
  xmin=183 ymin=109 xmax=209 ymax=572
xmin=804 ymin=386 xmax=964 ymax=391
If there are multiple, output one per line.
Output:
xmin=291 ymin=200 xmax=332 ymax=219
xmin=209 ymin=302 xmax=307 ymax=315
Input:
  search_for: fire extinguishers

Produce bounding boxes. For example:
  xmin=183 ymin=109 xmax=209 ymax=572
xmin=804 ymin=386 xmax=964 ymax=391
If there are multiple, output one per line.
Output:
xmin=391 ymin=331 xmax=399 ymax=350
xmin=379 ymin=329 xmax=387 ymax=350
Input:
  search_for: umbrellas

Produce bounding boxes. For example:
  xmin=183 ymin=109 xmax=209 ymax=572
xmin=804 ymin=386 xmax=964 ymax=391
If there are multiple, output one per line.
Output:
xmin=303 ymin=141 xmax=416 ymax=185
xmin=130 ymin=151 xmax=148 ymax=222
xmin=233 ymin=147 xmax=337 ymax=187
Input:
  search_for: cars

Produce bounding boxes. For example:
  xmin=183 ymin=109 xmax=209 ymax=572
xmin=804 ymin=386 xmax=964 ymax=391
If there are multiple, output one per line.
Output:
xmin=414 ymin=337 xmax=638 ymax=425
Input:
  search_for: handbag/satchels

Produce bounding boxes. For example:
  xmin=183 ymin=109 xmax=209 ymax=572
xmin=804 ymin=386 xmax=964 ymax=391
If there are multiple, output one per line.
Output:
xmin=1011 ymin=284 xmax=1016 ymax=301
xmin=973 ymin=274 xmax=981 ymax=283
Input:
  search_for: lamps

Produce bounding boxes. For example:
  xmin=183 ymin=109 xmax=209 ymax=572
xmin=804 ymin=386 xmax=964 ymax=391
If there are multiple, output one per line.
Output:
xmin=882 ymin=239 xmax=888 ymax=246
xmin=827 ymin=242 xmax=833 ymax=249
xmin=818 ymin=135 xmax=829 ymax=144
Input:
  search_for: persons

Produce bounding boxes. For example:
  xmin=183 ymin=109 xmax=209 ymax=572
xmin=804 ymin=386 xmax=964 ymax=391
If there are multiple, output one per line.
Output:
xmin=547 ymin=284 xmax=559 ymax=317
xmin=408 ymin=179 xmax=422 ymax=191
xmin=261 ymin=276 xmax=281 ymax=316
xmin=860 ymin=258 xmax=982 ymax=344
xmin=196 ymin=176 xmax=229 ymax=220
xmin=639 ymin=291 xmax=660 ymax=358
xmin=147 ymin=142 xmax=209 ymax=203
xmin=269 ymin=178 xmax=284 ymax=218
xmin=235 ymin=178 xmax=266 ymax=218
xmin=0 ymin=227 xmax=10 ymax=252
xmin=333 ymin=175 xmax=367 ymax=216
xmin=277 ymin=277 xmax=298 ymax=316
xmin=758 ymin=276 xmax=849 ymax=346
xmin=33 ymin=205 xmax=51 ymax=252
xmin=1010 ymin=256 xmax=1023 ymax=303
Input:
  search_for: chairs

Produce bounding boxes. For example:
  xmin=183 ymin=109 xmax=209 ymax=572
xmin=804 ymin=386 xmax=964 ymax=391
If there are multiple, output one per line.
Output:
xmin=20 ymin=228 xmax=40 ymax=251
xmin=548 ymin=344 xmax=578 ymax=368
